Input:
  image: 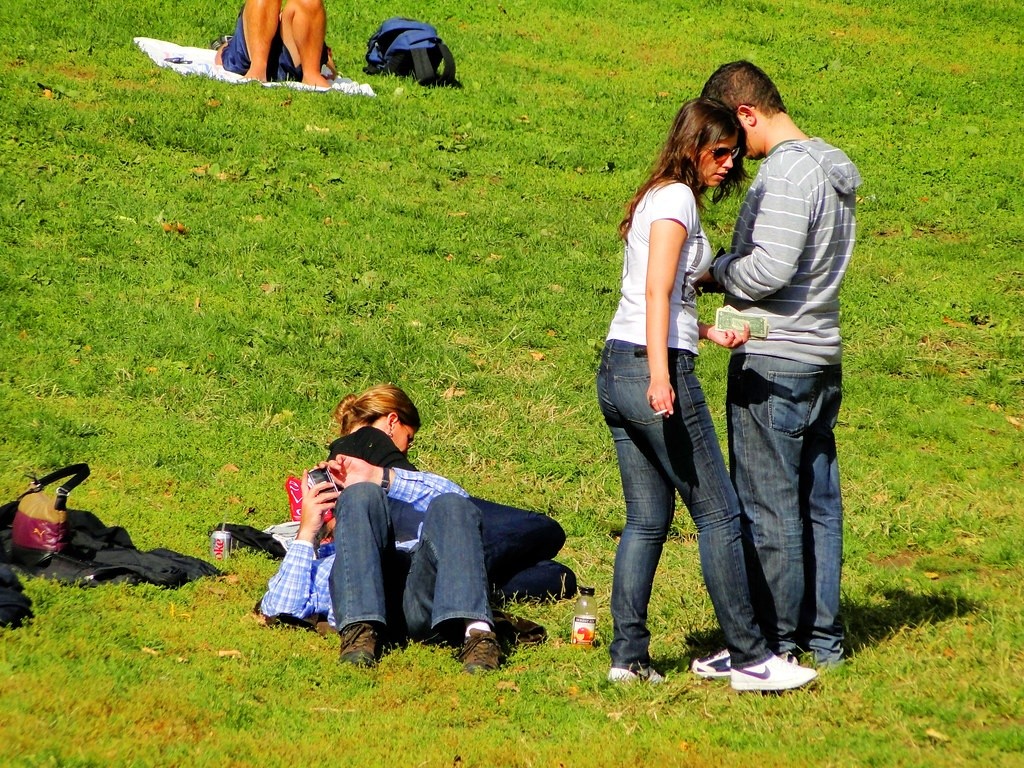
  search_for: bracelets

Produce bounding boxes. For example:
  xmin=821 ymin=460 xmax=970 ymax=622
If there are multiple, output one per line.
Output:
xmin=381 ymin=467 xmax=390 ymax=493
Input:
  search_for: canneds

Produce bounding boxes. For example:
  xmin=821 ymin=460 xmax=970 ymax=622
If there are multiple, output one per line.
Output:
xmin=210 ymin=531 xmax=232 ymax=560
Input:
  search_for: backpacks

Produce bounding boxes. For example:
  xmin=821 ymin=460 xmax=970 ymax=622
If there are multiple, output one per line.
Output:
xmin=363 ymin=18 xmax=456 ymax=86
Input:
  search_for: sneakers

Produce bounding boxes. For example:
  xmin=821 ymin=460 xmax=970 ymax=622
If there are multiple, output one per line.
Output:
xmin=692 ymin=644 xmax=797 ymax=676
xmin=458 ymin=628 xmax=499 ymax=676
xmin=608 ymin=665 xmax=663 ymax=684
xmin=730 ymin=655 xmax=818 ymax=690
xmin=339 ymin=623 xmax=377 ymax=665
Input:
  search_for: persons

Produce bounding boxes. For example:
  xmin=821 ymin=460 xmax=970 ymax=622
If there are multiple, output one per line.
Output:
xmin=214 ymin=0 xmax=338 ymax=91
xmin=692 ymin=62 xmax=861 ymax=677
xmin=596 ymin=95 xmax=819 ymax=694
xmin=260 ymin=384 xmax=578 ymax=676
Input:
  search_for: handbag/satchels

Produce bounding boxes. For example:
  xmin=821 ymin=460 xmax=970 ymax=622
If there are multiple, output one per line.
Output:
xmin=10 ymin=463 xmax=90 ymax=567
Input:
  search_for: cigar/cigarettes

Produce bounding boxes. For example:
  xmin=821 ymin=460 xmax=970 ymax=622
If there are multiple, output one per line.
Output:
xmin=654 ymin=410 xmax=667 ymax=417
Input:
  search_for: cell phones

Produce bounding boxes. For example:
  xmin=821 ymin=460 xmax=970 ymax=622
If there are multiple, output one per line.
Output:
xmin=308 ymin=468 xmax=338 ymax=504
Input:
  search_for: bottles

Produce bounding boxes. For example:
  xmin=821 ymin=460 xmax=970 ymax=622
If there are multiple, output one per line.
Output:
xmin=571 ymin=587 xmax=597 ymax=645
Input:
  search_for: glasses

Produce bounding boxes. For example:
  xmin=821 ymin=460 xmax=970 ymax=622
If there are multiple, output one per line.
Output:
xmin=702 ymin=144 xmax=740 ymax=161
